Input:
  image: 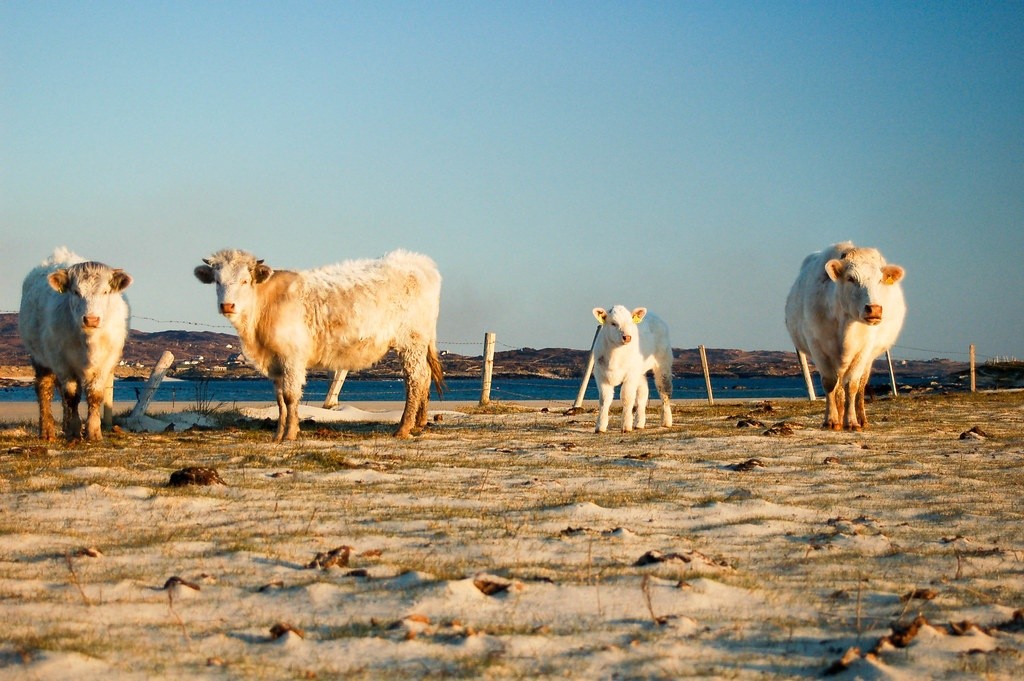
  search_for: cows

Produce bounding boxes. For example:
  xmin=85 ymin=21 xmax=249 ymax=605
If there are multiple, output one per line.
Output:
xmin=18 ymin=246 xmax=132 ymax=444
xmin=196 ymin=248 xmax=446 ymax=441
xmin=784 ymin=239 xmax=909 ymax=433
xmin=585 ymin=303 xmax=675 ymax=434
